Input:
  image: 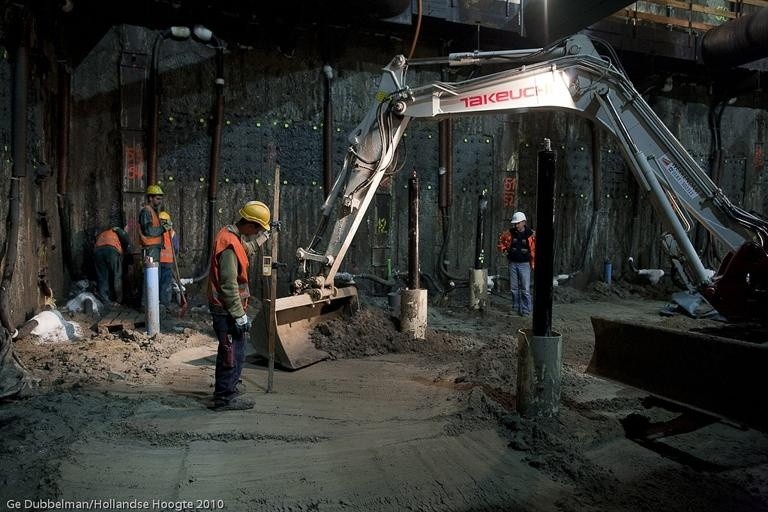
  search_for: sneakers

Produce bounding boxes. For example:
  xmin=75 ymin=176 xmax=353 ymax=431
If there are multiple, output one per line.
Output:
xmin=522 ymin=311 xmax=530 ymax=317
xmin=214 ymin=393 xmax=256 ymax=411
xmin=508 ymin=308 xmax=519 ymax=315
xmin=233 ymin=379 xmax=248 ymax=395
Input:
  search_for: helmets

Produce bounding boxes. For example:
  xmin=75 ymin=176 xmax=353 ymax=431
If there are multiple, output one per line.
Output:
xmin=239 ymin=200 xmax=273 ymax=231
xmin=510 ymin=210 xmax=527 ymax=224
xmin=147 ymin=184 xmax=164 ymax=196
xmin=158 ymin=210 xmax=170 ymax=220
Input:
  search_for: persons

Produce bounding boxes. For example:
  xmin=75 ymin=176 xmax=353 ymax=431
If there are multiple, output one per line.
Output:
xmin=158 ymin=211 xmax=179 ymax=307
xmin=497 ymin=212 xmax=535 ymax=316
xmin=94 ymin=227 xmax=129 ymax=304
xmin=207 ymin=200 xmax=282 ymax=412
xmin=136 ymin=185 xmax=172 ymax=313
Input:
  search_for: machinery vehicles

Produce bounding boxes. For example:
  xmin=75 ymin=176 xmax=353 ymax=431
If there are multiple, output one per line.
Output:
xmin=248 ymin=33 xmax=768 ymax=431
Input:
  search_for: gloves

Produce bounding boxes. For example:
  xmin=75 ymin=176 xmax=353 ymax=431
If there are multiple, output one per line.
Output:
xmin=235 ymin=313 xmax=251 ymax=332
xmin=163 ymin=220 xmax=174 ymax=232
xmin=264 ymin=220 xmax=281 ymax=238
